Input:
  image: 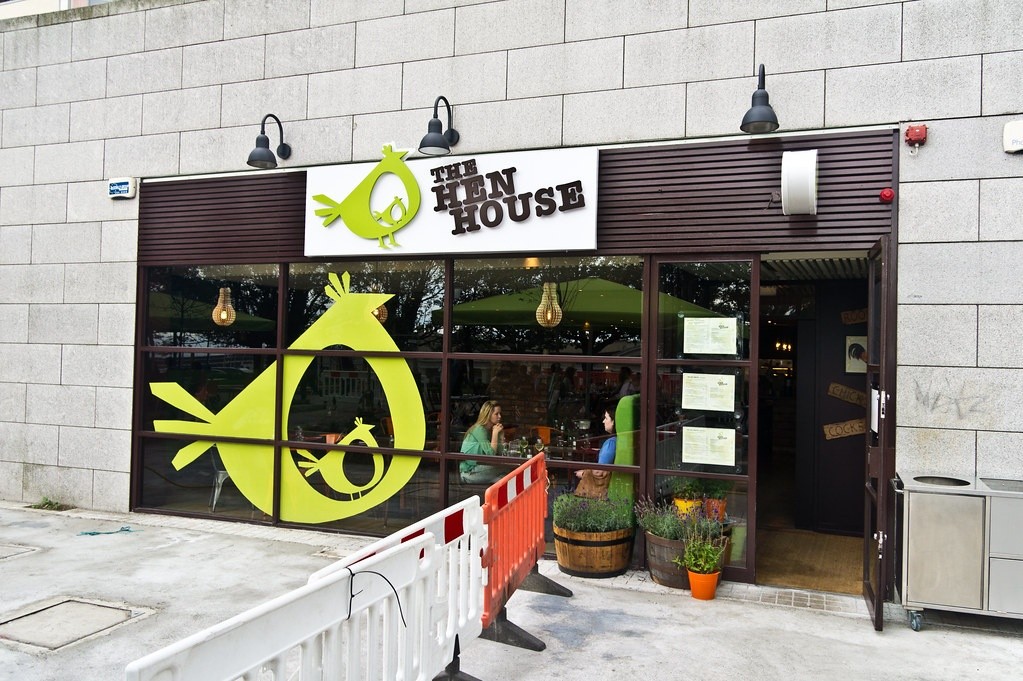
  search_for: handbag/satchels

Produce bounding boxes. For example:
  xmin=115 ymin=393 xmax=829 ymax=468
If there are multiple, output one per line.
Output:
xmin=575 ymin=469 xmax=610 ymax=502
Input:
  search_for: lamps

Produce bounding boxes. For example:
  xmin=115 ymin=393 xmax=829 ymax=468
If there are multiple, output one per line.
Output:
xmin=535 ymin=257 xmax=563 ymax=328
xmin=211 ymin=264 xmax=235 ymax=326
xmin=417 ymin=96 xmax=460 ymax=156
xmin=371 ymin=261 xmax=388 ymax=325
xmin=247 ymin=113 xmax=292 ymax=169
xmin=740 ymin=64 xmax=780 ymax=133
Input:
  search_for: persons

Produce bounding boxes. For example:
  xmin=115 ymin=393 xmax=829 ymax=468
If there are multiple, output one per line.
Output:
xmin=569 ymin=402 xmax=617 ymax=496
xmin=613 ymin=366 xmax=636 ymax=399
xmin=459 ymin=401 xmax=512 ymax=486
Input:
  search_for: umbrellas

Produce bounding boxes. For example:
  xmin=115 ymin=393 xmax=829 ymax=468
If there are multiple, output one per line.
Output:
xmin=431 ymin=275 xmax=749 ymax=420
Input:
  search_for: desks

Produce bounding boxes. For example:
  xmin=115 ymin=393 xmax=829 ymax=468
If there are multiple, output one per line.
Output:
xmin=546 ymin=446 xmax=599 ymax=491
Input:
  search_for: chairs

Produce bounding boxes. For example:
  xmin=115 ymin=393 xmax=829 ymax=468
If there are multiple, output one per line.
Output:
xmin=209 ymin=409 xmax=563 ymax=529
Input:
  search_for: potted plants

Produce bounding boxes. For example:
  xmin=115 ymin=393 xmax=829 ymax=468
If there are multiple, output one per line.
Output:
xmin=701 ymin=477 xmax=737 ymax=523
xmin=669 ymin=476 xmax=706 ymax=523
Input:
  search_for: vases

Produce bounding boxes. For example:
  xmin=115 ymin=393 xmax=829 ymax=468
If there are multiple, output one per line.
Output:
xmin=645 ymin=529 xmax=733 ymax=590
xmin=550 ymin=522 xmax=633 ymax=579
xmin=687 ymin=569 xmax=720 ymax=600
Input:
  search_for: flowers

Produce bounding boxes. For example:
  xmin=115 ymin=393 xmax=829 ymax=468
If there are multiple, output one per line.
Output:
xmin=671 ymin=509 xmax=732 ymax=572
xmin=553 ymin=491 xmax=633 ymax=530
xmin=634 ymin=487 xmax=740 ymax=540
